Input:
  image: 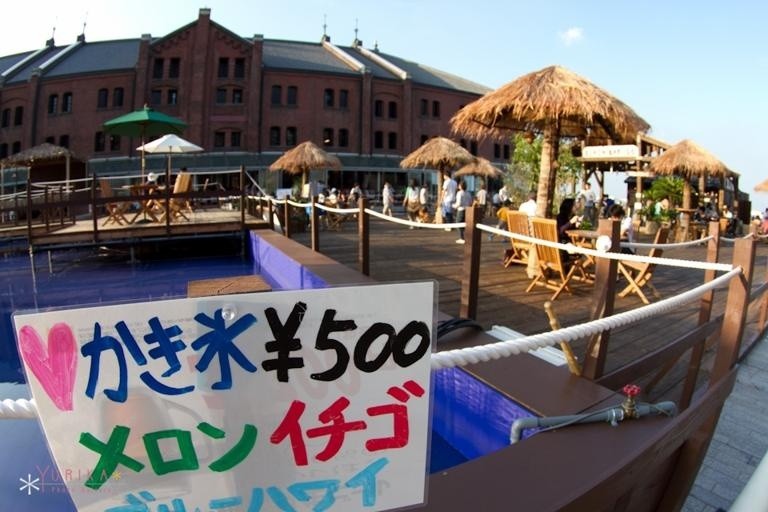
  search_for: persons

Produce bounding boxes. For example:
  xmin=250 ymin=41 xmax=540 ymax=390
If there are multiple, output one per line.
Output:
xmin=645 ymin=197 xmax=768 ymax=247
xmin=607 ymin=205 xmax=635 ymax=255
xmin=492 ymin=190 xmax=502 ymax=212
xmin=577 ymin=182 xmax=597 ymax=226
xmin=452 ymin=178 xmax=473 ymax=245
xmin=419 ymin=184 xmax=431 ymax=213
xmin=439 ymin=170 xmax=459 ymax=233
xmin=498 ymin=184 xmax=512 ymax=206
xmin=142 ymin=170 xmax=161 ymax=194
xmin=402 ymin=178 xmax=422 ymax=230
xmin=518 ymin=191 xmax=538 ymax=217
xmin=382 ymin=181 xmax=395 ymax=218
xmin=489 ymin=199 xmax=513 ymax=243
xmin=555 ymin=198 xmax=583 ymax=274
xmin=301 ymin=180 xmax=363 ymax=230
xmin=476 ymin=183 xmax=492 ymax=218
xmin=601 ymin=194 xmax=615 ymax=218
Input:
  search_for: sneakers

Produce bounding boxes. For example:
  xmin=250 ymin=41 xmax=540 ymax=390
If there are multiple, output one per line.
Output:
xmin=486 ymin=235 xmax=507 ymax=243
xmin=444 ymin=227 xmax=452 ymax=232
xmin=455 ymin=239 xmax=466 ymax=244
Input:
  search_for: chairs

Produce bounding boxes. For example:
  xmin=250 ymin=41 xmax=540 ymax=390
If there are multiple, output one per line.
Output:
xmin=502 ymin=210 xmax=530 ymax=269
xmin=619 ymin=227 xmax=672 ymax=303
xmin=98 ymin=179 xmax=130 ymax=226
xmin=525 ymin=218 xmax=573 ymax=296
xmin=154 ymin=172 xmax=192 ymax=222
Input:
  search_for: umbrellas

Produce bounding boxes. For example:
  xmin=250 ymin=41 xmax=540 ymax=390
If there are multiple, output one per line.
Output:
xmin=135 ymin=132 xmax=205 ymax=193
xmin=100 ymin=104 xmax=189 ymax=219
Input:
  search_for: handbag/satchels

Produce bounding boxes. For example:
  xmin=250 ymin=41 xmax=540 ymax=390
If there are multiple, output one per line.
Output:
xmin=407 ymin=202 xmax=420 ymax=210
